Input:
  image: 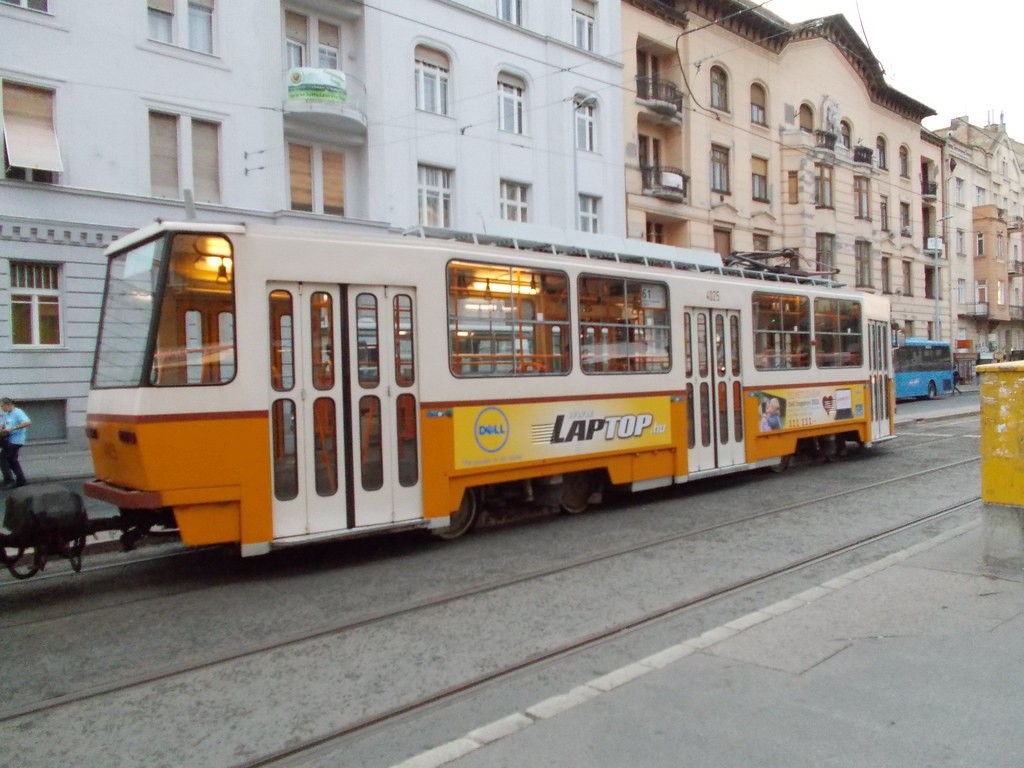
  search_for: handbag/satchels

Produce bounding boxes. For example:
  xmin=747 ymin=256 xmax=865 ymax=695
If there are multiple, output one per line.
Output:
xmin=0 ymin=433 xmax=7 ymax=448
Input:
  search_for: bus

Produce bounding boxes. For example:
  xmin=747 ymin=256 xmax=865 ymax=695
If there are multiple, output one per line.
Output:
xmin=892 ymin=337 xmax=953 ymax=400
xmin=85 ymin=214 xmax=898 ymax=559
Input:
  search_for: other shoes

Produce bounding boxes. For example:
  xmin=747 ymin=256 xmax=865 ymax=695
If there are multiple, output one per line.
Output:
xmin=13 ymin=480 xmax=26 ymax=488
xmin=2 ymin=479 xmax=14 ymax=487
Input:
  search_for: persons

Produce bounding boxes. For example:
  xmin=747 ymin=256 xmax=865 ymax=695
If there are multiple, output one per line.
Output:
xmin=952 ymin=366 xmax=961 ymax=395
xmin=0 ymin=396 xmax=33 ymax=490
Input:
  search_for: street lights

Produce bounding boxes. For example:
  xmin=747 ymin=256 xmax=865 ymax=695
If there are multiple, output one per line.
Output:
xmin=935 ymin=215 xmax=954 ymax=341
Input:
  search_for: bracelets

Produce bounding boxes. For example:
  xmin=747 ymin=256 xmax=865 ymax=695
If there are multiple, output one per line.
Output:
xmin=13 ymin=426 xmax=16 ymax=431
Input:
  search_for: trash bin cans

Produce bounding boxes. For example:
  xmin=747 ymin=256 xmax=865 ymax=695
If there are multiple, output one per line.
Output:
xmin=977 ymin=362 xmax=1024 ymax=506
